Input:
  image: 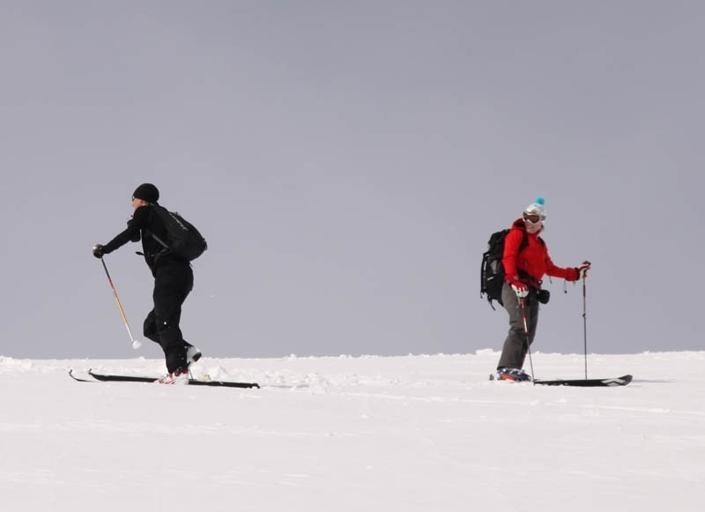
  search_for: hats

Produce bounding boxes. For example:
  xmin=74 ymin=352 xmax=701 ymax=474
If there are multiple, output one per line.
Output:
xmin=133 ymin=183 xmax=159 ymax=202
xmin=522 ymin=196 xmax=548 ymax=225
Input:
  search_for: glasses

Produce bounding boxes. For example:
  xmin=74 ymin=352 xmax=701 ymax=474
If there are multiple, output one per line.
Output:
xmin=523 ymin=211 xmax=539 ymax=224
xmin=132 ymin=195 xmax=135 ymax=200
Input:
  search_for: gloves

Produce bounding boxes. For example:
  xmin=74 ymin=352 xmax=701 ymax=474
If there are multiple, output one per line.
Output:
xmin=93 ymin=244 xmax=104 ymax=258
xmin=577 ymin=260 xmax=592 ymax=279
xmin=511 ymin=281 xmax=529 ymax=298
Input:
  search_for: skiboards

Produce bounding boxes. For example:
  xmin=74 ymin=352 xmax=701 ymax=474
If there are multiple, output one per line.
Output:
xmin=489 ymin=372 xmax=632 ymax=387
xmin=68 ymin=369 xmax=262 ymax=390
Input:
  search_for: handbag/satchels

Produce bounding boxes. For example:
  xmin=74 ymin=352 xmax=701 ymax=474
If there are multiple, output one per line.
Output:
xmin=540 ymin=289 xmax=550 ymax=304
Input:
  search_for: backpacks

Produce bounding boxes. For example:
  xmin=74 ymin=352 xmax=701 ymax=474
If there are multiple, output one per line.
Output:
xmin=480 ymin=229 xmax=528 ymax=299
xmin=159 ymin=211 xmax=207 ymax=260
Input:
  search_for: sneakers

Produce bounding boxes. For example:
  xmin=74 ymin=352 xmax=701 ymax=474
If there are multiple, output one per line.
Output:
xmin=496 ymin=368 xmax=532 ymax=383
xmin=152 ymin=346 xmax=201 ymax=385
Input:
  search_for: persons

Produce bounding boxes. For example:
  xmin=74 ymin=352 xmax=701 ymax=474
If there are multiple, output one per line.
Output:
xmin=93 ymin=181 xmax=203 ymax=387
xmin=496 ymin=196 xmax=592 ymax=384
